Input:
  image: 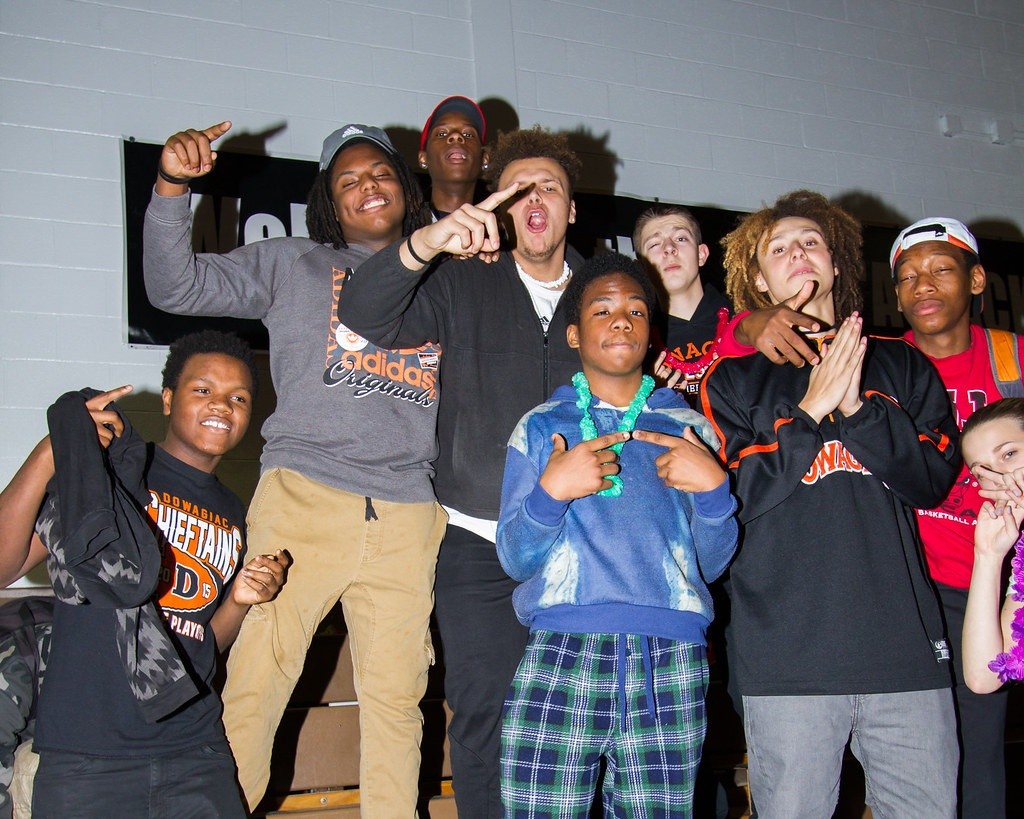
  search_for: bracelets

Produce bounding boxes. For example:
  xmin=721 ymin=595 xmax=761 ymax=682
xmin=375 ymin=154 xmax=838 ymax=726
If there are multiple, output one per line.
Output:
xmin=158 ymin=166 xmax=191 ymax=184
xmin=408 ymin=234 xmax=433 ymax=264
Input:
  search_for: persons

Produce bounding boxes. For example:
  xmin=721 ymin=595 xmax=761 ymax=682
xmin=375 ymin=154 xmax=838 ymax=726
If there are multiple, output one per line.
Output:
xmin=0 ymin=327 xmax=294 ymax=819
xmin=496 ymin=253 xmax=739 ymax=819
xmin=145 ymin=121 xmax=501 ymax=819
xmin=634 ymin=208 xmax=748 ymax=819
xmin=715 ymin=217 xmax=1023 ymax=819
xmin=962 ymin=396 xmax=1024 ymax=694
xmin=696 ymin=190 xmax=963 ymax=819
xmin=340 ymin=127 xmax=586 ymax=819
xmin=403 ymin=95 xmax=496 ymax=242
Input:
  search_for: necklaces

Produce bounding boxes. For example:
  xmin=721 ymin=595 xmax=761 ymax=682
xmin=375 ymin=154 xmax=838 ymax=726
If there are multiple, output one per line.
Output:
xmin=571 ymin=372 xmax=655 ymax=498
xmin=515 ymin=259 xmax=570 ymax=287
xmin=988 ymin=532 xmax=1024 ymax=682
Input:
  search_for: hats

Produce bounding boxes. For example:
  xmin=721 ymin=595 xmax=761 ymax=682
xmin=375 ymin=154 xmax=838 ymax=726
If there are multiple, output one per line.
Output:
xmin=318 ymin=124 xmax=398 ymax=171
xmin=890 ymin=217 xmax=985 ymax=315
xmin=419 ymin=94 xmax=487 ymax=148
